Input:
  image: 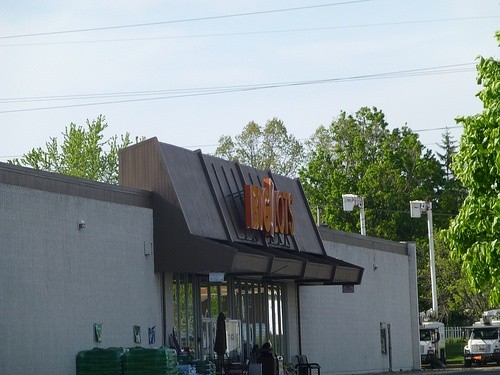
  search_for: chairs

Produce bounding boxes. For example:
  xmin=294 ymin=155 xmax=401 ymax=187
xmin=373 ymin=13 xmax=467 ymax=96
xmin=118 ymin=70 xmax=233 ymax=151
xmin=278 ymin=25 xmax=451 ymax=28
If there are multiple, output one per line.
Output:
xmin=295 ymin=355 xmax=321 ymax=375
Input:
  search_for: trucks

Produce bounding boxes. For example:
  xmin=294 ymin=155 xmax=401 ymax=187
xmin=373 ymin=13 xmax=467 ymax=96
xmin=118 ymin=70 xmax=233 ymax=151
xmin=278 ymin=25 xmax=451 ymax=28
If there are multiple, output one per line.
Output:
xmin=419 ymin=307 xmax=447 ymax=369
xmin=462 ymin=309 xmax=500 ymax=367
